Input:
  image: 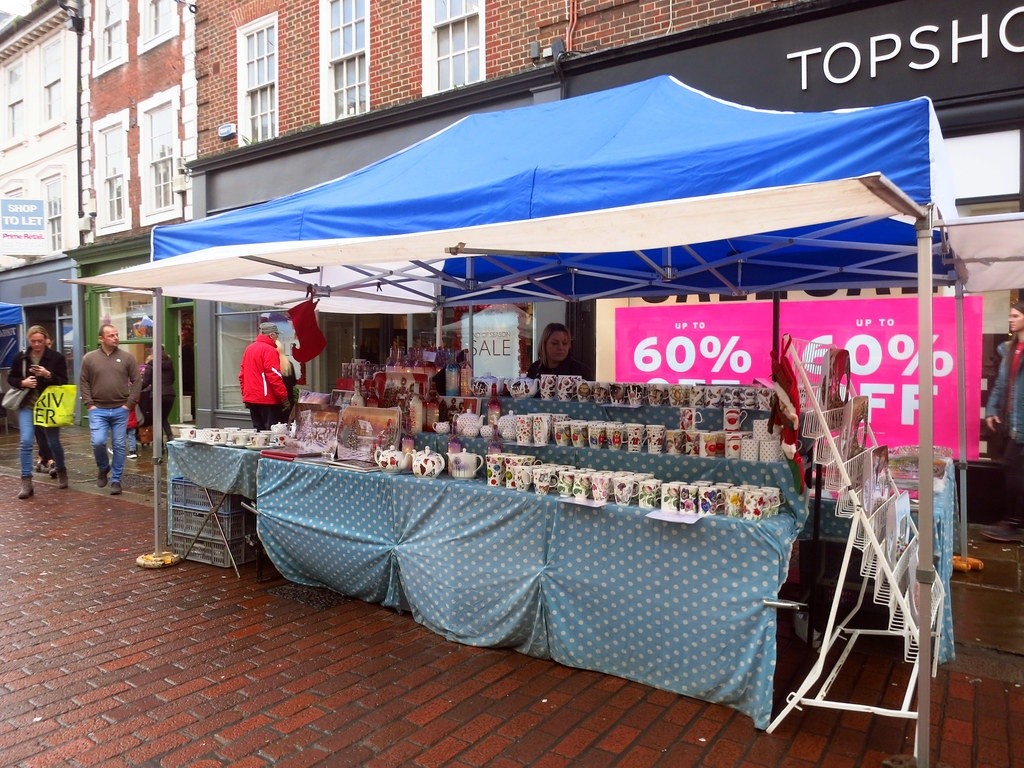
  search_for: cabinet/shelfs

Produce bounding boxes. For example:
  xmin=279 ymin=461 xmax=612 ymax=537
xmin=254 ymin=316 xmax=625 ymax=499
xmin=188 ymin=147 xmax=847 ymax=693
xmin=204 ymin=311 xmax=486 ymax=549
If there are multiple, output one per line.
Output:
xmin=766 ymin=334 xmax=945 ymax=754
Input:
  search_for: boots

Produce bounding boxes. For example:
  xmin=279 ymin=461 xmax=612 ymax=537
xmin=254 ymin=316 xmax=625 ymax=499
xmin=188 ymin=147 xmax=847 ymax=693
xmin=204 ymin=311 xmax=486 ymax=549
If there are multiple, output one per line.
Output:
xmin=18 ymin=475 xmax=33 ymax=499
xmin=56 ymin=467 xmax=68 ymax=489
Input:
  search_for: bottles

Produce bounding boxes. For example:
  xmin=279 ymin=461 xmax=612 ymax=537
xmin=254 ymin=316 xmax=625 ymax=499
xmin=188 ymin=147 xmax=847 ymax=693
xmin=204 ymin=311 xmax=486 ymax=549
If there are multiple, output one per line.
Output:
xmin=446 ymin=448 xmax=484 ymax=480
xmin=367 ymin=386 xmax=378 ymax=407
xmin=488 ymin=425 xmax=503 ymax=453
xmin=446 ymin=420 xmax=461 ymax=476
xmin=487 ymin=382 xmax=501 ymax=435
xmin=401 ymin=417 xmax=415 ymax=473
xmin=350 ymin=380 xmax=365 ymax=407
xmin=386 ymin=347 xmax=474 ymax=433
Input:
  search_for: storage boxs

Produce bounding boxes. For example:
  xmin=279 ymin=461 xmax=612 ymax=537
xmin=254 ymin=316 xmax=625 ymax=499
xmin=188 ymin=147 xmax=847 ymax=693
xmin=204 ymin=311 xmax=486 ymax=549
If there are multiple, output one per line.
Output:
xmin=170 ymin=476 xmax=251 ymax=513
xmin=171 ymin=507 xmax=257 ymax=539
xmin=172 ymin=534 xmax=258 ymax=568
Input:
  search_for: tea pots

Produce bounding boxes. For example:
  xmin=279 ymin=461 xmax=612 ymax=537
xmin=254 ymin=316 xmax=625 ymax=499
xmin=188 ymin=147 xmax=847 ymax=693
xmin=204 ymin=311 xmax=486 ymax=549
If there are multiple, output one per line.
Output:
xmin=453 ymin=408 xmax=485 ymax=437
xmin=374 ymin=445 xmax=410 ymax=474
xmin=469 ymin=371 xmax=540 ymax=398
xmin=491 ymin=410 xmax=516 ymax=440
xmin=270 ymin=422 xmax=288 ymax=436
xmin=411 ymin=445 xmax=445 ymax=479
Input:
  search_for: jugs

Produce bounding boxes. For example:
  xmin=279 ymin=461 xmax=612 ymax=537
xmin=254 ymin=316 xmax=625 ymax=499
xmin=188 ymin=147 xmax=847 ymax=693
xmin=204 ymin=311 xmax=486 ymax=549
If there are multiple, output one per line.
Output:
xmin=431 ymin=421 xmax=450 ymax=434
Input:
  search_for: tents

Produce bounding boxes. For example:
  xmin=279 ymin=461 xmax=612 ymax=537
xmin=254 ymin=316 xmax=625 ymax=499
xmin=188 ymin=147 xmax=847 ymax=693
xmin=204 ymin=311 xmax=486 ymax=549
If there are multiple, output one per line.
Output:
xmin=150 ymin=75 xmax=967 ymax=765
xmin=0 ymin=302 xmax=27 ymax=377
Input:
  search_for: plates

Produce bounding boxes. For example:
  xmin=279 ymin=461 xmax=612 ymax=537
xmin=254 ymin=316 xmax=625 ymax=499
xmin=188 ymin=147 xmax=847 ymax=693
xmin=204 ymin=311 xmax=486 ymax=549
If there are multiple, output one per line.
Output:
xmin=173 ymin=437 xmax=285 ymax=450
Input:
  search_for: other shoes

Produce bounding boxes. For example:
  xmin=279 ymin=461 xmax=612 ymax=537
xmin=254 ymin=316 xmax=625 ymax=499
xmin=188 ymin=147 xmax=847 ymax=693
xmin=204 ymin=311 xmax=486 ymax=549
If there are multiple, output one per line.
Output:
xmin=978 ymin=519 xmax=1024 ymax=542
xmin=127 ymin=450 xmax=138 ymax=458
xmin=111 ymin=482 xmax=122 ymax=494
xmin=49 ymin=462 xmax=57 ymax=477
xmin=97 ymin=464 xmax=111 ymax=486
xmin=36 ymin=462 xmax=49 ymax=473
xmin=108 ymin=448 xmax=115 ymax=455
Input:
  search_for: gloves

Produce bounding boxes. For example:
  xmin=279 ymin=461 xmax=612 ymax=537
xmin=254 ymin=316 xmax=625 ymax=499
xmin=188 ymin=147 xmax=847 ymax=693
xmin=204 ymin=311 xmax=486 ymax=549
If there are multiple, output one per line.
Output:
xmin=282 ymin=399 xmax=291 ymax=411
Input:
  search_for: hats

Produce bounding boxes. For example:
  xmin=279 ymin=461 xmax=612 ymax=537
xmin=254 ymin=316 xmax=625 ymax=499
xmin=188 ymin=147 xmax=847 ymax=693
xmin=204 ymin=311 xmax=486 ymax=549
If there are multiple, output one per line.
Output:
xmin=259 ymin=322 xmax=282 ymax=334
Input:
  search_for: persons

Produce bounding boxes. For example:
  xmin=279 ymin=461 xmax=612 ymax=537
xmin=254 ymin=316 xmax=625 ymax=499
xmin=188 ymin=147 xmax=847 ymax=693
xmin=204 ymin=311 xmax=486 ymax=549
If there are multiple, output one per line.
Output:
xmin=7 ymin=325 xmax=68 ymax=498
xmin=526 ymin=323 xmax=593 ymax=381
xmin=34 ymin=334 xmax=57 ymax=479
xmin=80 ymin=325 xmax=141 ymax=495
xmin=140 ymin=346 xmax=176 ymax=455
xmin=981 ymin=300 xmax=1024 ymax=541
xmin=238 ymin=323 xmax=290 ymax=431
xmin=275 ymin=340 xmax=296 ymax=422
xmin=108 ymin=408 xmax=138 ymax=458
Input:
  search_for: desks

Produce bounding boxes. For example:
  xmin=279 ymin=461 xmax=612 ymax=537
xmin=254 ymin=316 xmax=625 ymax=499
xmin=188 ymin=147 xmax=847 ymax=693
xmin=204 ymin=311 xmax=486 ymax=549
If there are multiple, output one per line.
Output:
xmin=254 ymin=456 xmax=804 ymax=727
xmin=803 ymin=455 xmax=958 ymax=652
xmin=162 ymin=441 xmax=263 ymax=545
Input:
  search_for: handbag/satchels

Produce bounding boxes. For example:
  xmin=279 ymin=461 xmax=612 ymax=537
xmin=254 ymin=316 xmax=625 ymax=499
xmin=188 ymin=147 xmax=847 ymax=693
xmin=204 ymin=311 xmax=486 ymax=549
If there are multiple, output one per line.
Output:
xmin=1 ymin=350 xmax=30 ymax=410
xmin=127 ymin=403 xmax=145 ymax=428
xmin=33 ymin=375 xmax=78 ymax=427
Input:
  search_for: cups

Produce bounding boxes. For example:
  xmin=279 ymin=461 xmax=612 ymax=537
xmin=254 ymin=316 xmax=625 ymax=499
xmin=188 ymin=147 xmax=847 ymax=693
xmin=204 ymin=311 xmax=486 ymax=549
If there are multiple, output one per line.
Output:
xmin=516 ymin=407 xmax=783 ymax=463
xmin=485 ymin=453 xmax=786 ymax=522
xmin=540 ymin=374 xmax=775 ymax=412
xmin=179 ymin=427 xmax=273 ymax=445
xmin=342 ymin=359 xmax=385 ymax=380
xmin=481 ymin=425 xmax=494 ymax=438
